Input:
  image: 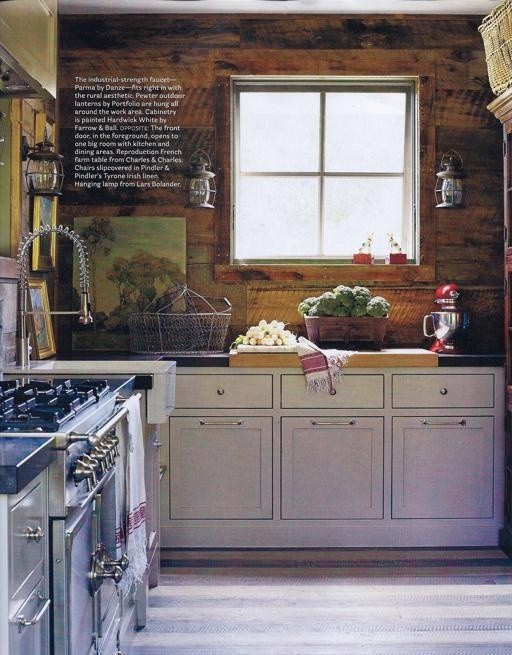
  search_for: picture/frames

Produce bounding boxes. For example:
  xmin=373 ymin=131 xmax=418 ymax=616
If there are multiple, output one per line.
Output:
xmin=26 ymin=111 xmax=59 ymax=360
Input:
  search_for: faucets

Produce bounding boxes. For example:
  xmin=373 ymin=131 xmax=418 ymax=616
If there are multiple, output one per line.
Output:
xmin=17 ymin=224 xmax=93 ymax=362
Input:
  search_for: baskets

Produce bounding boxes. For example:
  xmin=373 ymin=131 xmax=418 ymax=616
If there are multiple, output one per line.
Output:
xmin=126 ymin=282 xmax=232 ymax=355
xmin=477 ymin=1 xmax=512 ymax=96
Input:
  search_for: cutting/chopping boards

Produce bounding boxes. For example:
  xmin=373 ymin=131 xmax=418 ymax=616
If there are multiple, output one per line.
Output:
xmin=237 ymin=344 xmax=298 ymax=352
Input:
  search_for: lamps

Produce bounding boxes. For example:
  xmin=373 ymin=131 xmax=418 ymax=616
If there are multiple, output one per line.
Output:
xmin=432 ymin=146 xmax=468 ymax=210
xmin=22 ymin=127 xmax=63 ymax=196
xmin=181 ymin=148 xmax=217 ymax=210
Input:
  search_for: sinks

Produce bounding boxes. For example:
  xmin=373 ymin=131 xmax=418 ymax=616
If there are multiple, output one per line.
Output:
xmin=6 ymin=359 xmax=176 ymax=423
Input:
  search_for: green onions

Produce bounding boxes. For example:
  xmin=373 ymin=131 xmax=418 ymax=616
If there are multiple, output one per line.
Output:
xmin=228 ymin=320 xmax=296 ymax=351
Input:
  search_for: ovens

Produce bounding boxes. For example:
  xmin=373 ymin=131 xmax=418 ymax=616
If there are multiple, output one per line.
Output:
xmin=49 ymin=472 xmax=121 ymax=655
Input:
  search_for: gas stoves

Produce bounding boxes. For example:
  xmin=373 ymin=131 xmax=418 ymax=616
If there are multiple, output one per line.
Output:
xmin=0 ymin=378 xmax=121 ymax=519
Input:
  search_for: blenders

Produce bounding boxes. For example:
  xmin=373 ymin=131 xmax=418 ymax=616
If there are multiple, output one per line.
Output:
xmin=423 ymin=283 xmax=471 ymax=355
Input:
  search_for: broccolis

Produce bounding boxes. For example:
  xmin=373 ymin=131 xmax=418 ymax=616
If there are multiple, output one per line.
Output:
xmin=297 ymin=284 xmax=390 ymax=318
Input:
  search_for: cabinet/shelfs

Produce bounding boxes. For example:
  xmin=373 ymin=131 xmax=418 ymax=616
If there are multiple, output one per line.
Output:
xmin=6 ymin=467 xmax=50 ymax=655
xmin=151 ymin=374 xmax=499 ymax=548
